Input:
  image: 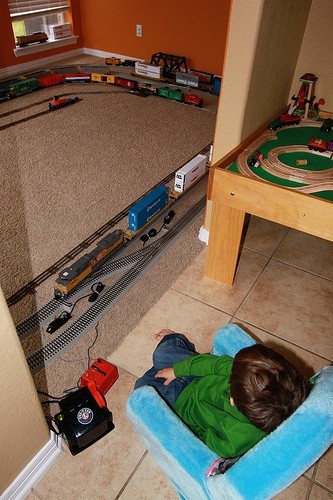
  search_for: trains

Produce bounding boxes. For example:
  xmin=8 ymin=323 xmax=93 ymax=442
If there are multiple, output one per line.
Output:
xmin=52 ymin=153 xmax=207 ymax=300
xmin=0 ymin=72 xmax=204 ymax=108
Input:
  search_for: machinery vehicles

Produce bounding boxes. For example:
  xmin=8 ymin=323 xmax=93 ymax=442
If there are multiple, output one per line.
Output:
xmin=16 ymin=31 xmax=48 ymax=47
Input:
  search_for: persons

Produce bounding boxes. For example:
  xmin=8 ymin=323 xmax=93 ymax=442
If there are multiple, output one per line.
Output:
xmin=133 ymin=328 xmax=306 ymax=458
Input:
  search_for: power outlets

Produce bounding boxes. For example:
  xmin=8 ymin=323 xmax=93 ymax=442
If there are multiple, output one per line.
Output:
xmin=136 ymin=25 xmax=142 ymax=37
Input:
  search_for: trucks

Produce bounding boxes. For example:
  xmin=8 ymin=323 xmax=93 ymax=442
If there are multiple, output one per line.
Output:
xmin=267 ymin=114 xmax=303 ymax=131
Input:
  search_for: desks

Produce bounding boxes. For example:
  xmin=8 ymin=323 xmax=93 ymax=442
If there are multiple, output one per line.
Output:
xmin=203 ymin=104 xmax=333 ymax=287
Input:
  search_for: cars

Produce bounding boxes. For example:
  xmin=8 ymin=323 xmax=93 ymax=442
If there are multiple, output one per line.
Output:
xmin=307 ymin=137 xmax=327 ymax=152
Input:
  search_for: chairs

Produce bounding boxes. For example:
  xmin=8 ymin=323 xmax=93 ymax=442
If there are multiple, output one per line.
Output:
xmin=125 ymin=323 xmax=333 ymax=499
